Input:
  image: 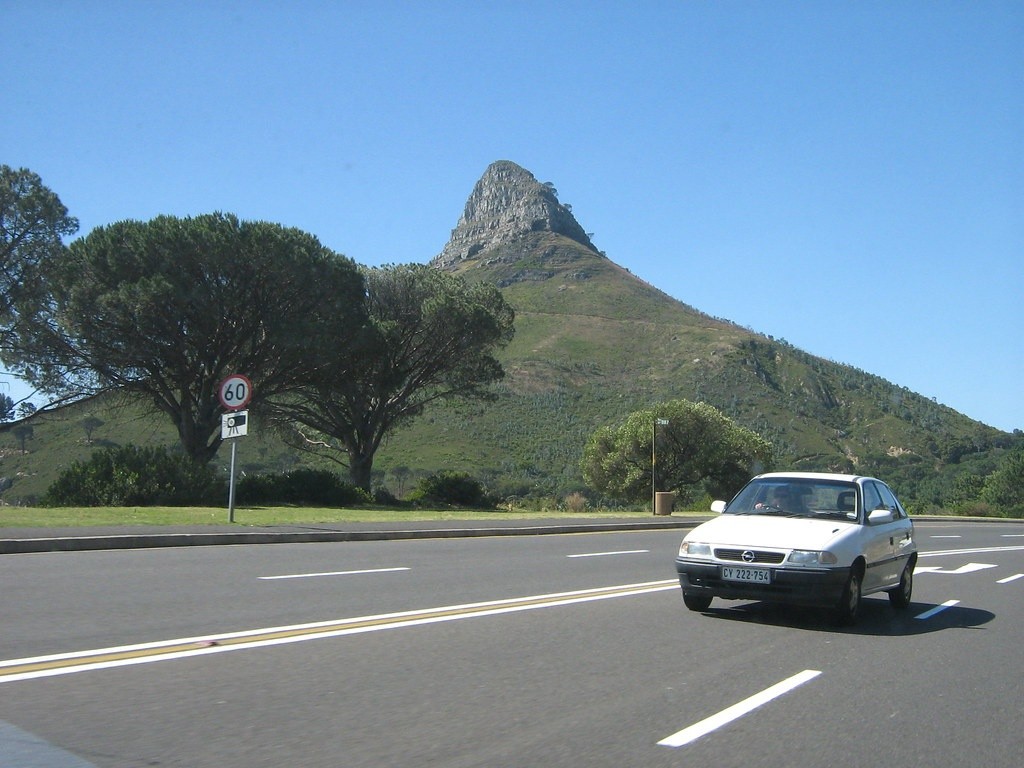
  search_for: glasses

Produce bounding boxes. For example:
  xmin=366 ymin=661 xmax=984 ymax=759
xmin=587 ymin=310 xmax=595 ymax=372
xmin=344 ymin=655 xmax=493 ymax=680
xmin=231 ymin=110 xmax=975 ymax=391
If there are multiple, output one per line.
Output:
xmin=774 ymin=493 xmax=789 ymax=500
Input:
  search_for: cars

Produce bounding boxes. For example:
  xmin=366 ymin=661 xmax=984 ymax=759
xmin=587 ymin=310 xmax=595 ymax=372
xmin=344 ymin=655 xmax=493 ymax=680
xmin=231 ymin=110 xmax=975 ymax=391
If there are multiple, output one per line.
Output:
xmin=675 ymin=470 xmax=919 ymax=621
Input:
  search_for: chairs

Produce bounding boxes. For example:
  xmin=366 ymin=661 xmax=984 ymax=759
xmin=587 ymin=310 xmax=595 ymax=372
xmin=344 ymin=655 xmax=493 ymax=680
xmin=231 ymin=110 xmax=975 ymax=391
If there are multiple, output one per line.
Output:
xmin=837 ymin=492 xmax=855 ymax=512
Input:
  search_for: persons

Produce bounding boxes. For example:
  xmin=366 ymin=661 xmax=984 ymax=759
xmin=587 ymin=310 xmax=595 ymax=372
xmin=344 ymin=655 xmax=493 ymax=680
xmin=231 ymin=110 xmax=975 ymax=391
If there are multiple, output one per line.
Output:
xmin=756 ymin=486 xmax=792 ymax=512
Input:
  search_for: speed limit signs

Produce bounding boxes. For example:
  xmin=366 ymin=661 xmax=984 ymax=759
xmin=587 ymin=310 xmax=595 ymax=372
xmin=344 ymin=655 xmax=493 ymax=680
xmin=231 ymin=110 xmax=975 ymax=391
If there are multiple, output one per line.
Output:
xmin=218 ymin=374 xmax=252 ymax=410
